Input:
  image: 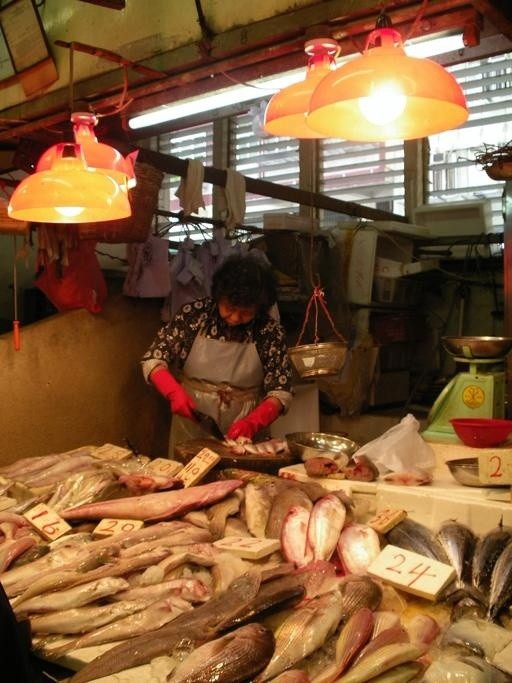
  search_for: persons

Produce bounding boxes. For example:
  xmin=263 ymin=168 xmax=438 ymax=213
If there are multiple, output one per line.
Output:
xmin=0 ymin=579 xmax=37 ymax=683
xmin=139 ymin=253 xmax=296 ymax=464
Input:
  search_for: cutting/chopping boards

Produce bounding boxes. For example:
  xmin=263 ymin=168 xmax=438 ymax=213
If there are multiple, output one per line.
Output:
xmin=172 ymin=439 xmax=302 ymax=473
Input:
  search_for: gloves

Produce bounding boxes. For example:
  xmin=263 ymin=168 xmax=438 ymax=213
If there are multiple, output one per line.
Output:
xmin=228 ymin=399 xmax=279 ymax=441
xmin=149 ymin=367 xmax=197 ymax=420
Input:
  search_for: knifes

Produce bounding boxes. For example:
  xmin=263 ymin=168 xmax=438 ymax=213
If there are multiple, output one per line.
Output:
xmin=189 ymin=408 xmax=226 ymax=442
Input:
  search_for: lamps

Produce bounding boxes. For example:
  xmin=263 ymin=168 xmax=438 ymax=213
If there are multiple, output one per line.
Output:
xmin=262 ymin=2 xmax=467 ymax=143
xmin=11 ymin=43 xmax=137 ymax=224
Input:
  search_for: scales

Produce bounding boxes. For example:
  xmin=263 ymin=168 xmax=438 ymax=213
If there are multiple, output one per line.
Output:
xmin=420 ymin=336 xmax=512 ymax=445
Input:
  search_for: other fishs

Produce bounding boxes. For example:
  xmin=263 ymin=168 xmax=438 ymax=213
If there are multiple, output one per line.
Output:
xmin=0 ymin=431 xmax=512 ymax=683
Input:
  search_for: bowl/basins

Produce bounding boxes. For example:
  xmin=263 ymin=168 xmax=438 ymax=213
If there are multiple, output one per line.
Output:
xmin=448 ymin=418 xmax=512 ymax=448
xmin=445 ymin=458 xmax=483 ymax=488
xmin=283 ymin=432 xmax=360 ymax=464
